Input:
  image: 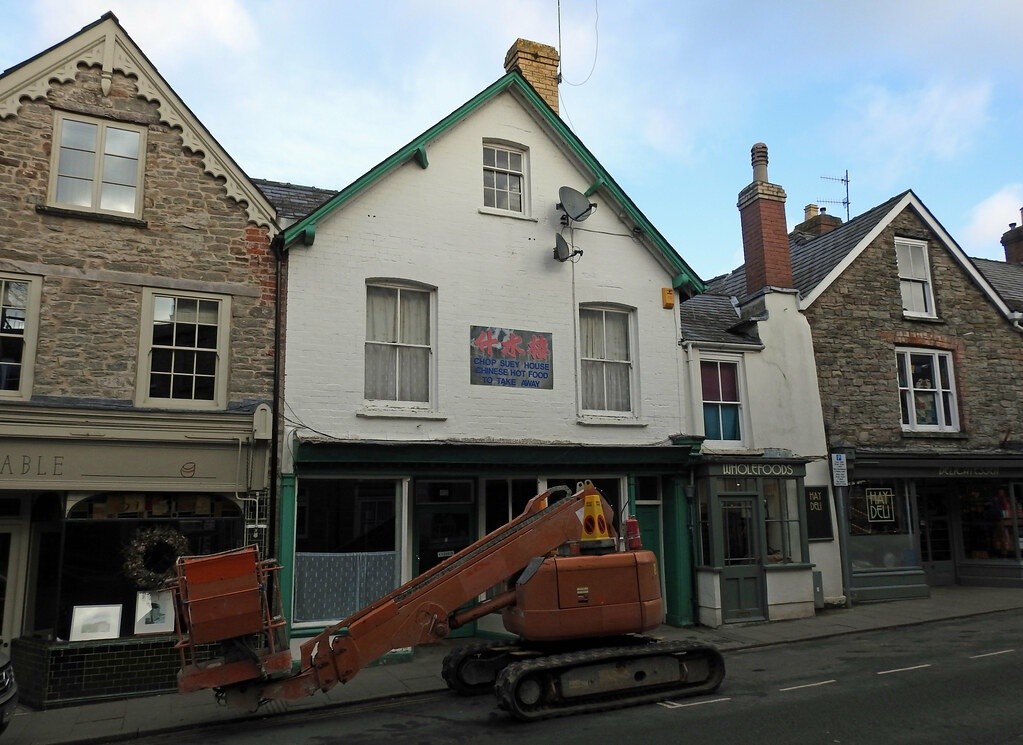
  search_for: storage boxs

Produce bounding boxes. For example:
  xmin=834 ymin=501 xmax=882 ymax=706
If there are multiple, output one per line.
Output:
xmin=179 ymin=548 xmax=263 ymax=642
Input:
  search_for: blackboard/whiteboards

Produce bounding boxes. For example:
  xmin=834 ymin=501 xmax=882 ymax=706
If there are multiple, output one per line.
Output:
xmin=803 ymin=485 xmax=835 ymax=541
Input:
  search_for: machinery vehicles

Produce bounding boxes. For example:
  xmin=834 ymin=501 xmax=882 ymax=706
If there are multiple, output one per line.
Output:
xmin=154 ymin=478 xmax=727 ymax=723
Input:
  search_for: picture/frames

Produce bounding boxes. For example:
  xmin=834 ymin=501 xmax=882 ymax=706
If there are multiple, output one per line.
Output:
xmin=133 ymin=589 xmax=176 ymax=635
xmin=68 ymin=603 xmax=122 ymax=641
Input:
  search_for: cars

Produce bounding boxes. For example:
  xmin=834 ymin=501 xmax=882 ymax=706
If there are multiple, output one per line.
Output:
xmin=0 ymin=649 xmax=17 ymax=734
